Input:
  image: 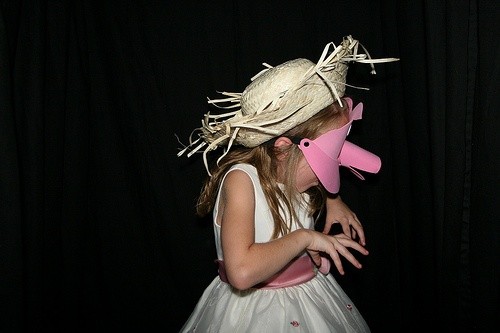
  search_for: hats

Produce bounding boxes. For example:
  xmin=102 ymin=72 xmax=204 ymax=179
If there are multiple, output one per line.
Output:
xmin=176 ymin=35 xmax=401 ymax=178
xmin=272 ymin=96 xmax=382 ymax=195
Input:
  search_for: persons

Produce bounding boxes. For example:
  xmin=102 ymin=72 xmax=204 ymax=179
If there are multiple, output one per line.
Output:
xmin=176 ymin=35 xmax=401 ymax=333
